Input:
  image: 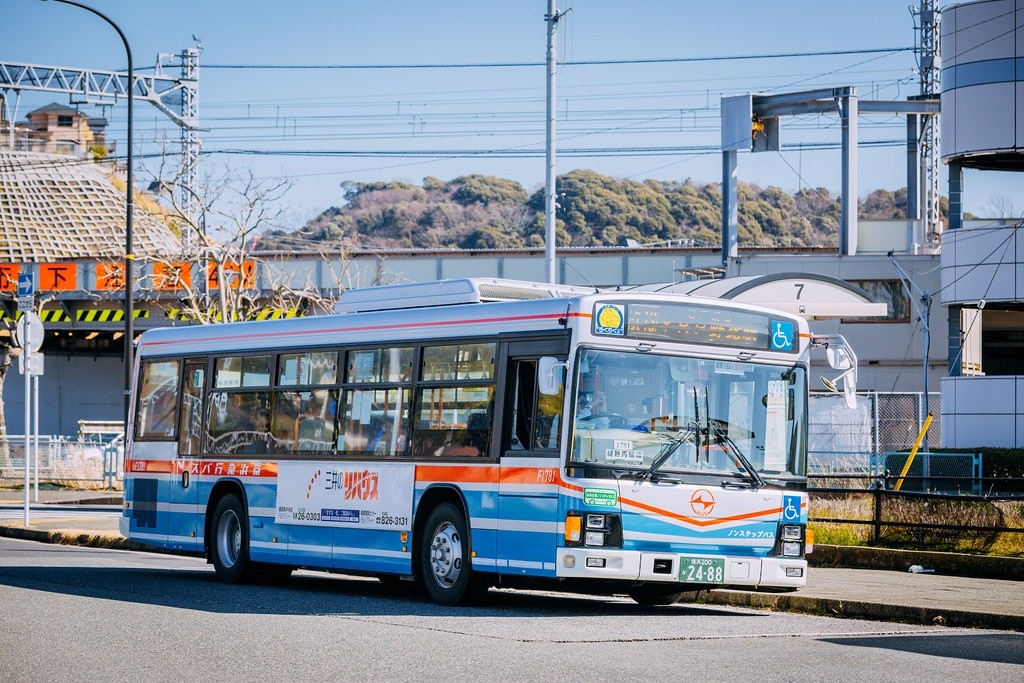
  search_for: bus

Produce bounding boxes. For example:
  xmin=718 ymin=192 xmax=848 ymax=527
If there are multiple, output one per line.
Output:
xmin=118 ymin=275 xmax=861 ymax=608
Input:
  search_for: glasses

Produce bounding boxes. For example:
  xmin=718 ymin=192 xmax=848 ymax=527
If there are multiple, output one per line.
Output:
xmin=578 ymin=400 xmax=588 ymax=406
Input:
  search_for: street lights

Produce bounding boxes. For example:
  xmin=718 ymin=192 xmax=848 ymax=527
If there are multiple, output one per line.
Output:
xmin=149 ymin=180 xmax=210 ymax=312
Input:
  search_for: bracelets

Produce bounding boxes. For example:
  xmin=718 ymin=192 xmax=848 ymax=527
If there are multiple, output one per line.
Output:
xmin=585 ymin=404 xmax=592 ymax=409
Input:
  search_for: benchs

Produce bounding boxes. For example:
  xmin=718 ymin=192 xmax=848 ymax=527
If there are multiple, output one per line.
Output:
xmin=293 ymin=415 xmax=337 ymax=452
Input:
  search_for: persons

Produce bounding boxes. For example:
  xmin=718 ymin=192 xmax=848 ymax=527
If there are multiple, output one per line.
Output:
xmin=548 ymin=389 xmax=612 ymax=447
